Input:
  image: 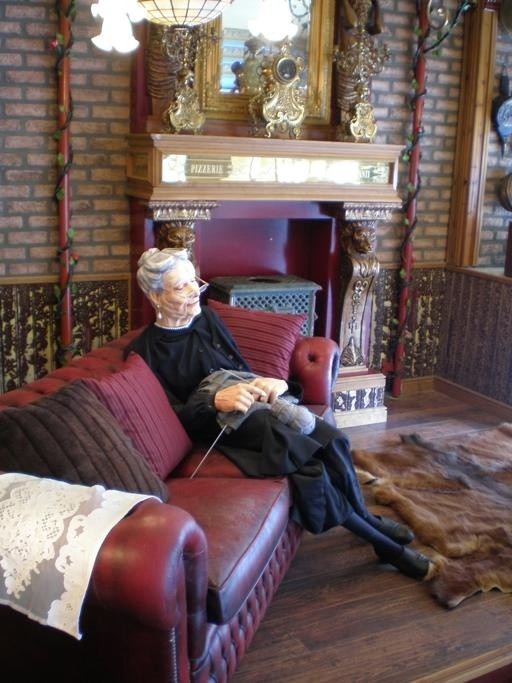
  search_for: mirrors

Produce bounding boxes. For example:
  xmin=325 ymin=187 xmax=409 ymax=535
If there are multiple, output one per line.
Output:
xmin=195 ymin=0 xmax=338 ymax=125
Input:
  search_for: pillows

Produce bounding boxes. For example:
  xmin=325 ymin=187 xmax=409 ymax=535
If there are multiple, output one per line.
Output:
xmin=82 ymin=352 xmax=192 ymax=481
xmin=207 ymin=297 xmax=305 ymax=398
xmin=2 ymin=378 xmax=169 ymax=504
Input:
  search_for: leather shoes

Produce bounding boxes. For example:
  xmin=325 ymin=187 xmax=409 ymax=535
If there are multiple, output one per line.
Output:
xmin=375 ymin=512 xmax=413 ymax=540
xmin=386 ymin=545 xmax=431 ymax=580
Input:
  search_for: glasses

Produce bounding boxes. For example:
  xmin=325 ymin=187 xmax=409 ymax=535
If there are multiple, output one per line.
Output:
xmin=162 ymin=275 xmax=210 ymax=299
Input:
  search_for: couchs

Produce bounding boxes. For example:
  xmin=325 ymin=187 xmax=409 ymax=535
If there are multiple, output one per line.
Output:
xmin=1 ymin=323 xmax=339 ymax=683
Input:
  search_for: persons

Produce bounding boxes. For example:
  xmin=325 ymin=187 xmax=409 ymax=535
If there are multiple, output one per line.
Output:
xmin=124 ymin=246 xmax=433 ymax=584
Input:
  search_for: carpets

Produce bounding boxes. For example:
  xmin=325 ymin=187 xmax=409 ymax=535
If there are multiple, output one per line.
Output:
xmin=347 ymin=423 xmax=512 ymax=612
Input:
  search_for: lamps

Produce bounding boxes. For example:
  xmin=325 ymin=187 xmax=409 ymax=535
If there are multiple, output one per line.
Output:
xmin=138 ymin=0 xmax=227 ymax=136
